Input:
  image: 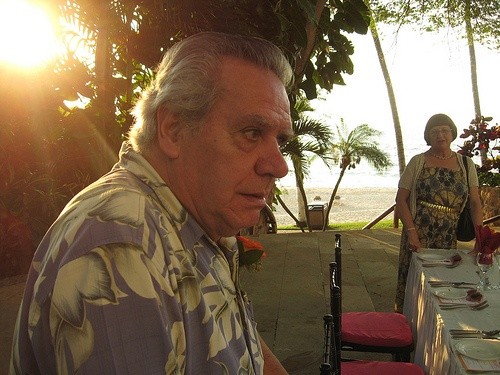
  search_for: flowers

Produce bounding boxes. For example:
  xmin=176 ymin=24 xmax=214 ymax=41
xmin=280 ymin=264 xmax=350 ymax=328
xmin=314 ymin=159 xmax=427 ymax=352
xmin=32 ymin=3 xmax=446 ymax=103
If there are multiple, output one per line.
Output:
xmin=233 ymin=234 xmax=267 ymax=276
xmin=473 ymin=223 xmax=500 ymax=267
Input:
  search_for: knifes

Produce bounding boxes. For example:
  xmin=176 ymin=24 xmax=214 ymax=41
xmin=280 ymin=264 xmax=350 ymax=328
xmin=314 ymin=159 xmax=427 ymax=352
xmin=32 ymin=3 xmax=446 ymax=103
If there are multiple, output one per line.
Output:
xmin=428 ymin=280 xmax=475 ymax=289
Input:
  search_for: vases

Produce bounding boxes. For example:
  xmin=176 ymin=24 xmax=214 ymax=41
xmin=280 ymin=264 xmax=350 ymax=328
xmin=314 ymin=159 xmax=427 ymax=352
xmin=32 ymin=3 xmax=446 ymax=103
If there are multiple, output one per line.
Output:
xmin=477 ymin=266 xmax=491 ymax=294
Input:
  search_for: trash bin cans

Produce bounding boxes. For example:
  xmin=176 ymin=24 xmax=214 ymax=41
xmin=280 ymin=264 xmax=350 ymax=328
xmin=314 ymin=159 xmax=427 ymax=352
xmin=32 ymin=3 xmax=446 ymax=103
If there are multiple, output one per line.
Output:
xmin=307 ymin=201 xmax=328 ymax=229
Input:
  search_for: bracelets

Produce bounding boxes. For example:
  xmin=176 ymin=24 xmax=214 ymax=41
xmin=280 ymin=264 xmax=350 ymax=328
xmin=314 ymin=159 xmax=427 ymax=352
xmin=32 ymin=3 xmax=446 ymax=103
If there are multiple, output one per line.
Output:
xmin=407 ymin=228 xmax=415 ymax=231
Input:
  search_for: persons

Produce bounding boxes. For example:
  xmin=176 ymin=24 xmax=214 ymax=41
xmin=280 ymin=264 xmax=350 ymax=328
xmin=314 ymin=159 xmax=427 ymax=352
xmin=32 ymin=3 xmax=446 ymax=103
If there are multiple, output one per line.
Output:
xmin=395 ymin=114 xmax=483 ymax=312
xmin=9 ymin=32 xmax=294 ymax=375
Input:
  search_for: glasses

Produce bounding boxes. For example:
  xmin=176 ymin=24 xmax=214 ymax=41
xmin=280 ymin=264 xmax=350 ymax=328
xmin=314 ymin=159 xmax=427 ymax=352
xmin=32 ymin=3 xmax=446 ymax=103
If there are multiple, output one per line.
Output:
xmin=429 ymin=128 xmax=452 ymax=137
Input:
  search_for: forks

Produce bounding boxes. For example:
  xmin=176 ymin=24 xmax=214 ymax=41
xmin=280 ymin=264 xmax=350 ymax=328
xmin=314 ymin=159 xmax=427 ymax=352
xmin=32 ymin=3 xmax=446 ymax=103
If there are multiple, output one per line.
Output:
xmin=421 ymin=264 xmax=460 ymax=269
xmin=449 ymin=328 xmax=500 ymax=339
xmin=439 ymin=300 xmax=490 ymax=310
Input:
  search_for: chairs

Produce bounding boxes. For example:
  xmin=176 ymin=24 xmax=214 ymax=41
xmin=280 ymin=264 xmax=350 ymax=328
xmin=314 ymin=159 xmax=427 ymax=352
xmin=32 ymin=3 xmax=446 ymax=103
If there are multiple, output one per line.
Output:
xmin=319 ymin=314 xmax=425 ymax=375
xmin=328 ymin=234 xmax=416 ymax=362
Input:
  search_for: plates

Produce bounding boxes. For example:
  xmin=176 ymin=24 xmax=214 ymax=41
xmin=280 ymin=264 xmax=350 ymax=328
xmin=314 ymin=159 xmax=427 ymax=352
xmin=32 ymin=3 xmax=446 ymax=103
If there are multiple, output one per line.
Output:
xmin=455 ymin=339 xmax=500 ymax=361
xmin=417 ymin=254 xmax=447 ymax=262
xmin=431 ymin=288 xmax=467 ymax=299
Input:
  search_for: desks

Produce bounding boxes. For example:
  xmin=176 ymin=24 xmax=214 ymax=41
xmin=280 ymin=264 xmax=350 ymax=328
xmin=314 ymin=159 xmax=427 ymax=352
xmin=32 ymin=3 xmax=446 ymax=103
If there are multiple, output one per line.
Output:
xmin=403 ymin=247 xmax=500 ymax=375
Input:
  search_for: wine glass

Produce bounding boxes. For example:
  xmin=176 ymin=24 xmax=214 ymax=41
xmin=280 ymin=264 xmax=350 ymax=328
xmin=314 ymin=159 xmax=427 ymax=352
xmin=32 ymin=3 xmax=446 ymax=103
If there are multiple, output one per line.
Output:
xmin=477 ymin=252 xmax=493 ymax=291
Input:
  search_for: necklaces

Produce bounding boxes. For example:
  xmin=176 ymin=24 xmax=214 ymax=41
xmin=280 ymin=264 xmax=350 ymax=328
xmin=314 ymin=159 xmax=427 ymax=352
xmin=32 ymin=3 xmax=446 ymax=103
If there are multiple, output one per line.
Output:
xmin=431 ymin=150 xmax=453 ymax=159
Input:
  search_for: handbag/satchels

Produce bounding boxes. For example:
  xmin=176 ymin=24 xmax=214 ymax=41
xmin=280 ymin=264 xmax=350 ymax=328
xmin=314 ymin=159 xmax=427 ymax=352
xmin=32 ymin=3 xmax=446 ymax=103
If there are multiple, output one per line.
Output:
xmin=456 ymin=154 xmax=475 ymax=242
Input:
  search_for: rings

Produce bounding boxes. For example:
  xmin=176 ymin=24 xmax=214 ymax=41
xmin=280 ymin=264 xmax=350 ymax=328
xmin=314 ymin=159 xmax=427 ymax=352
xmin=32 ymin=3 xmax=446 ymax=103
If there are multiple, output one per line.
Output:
xmin=410 ymin=249 xmax=412 ymax=251
